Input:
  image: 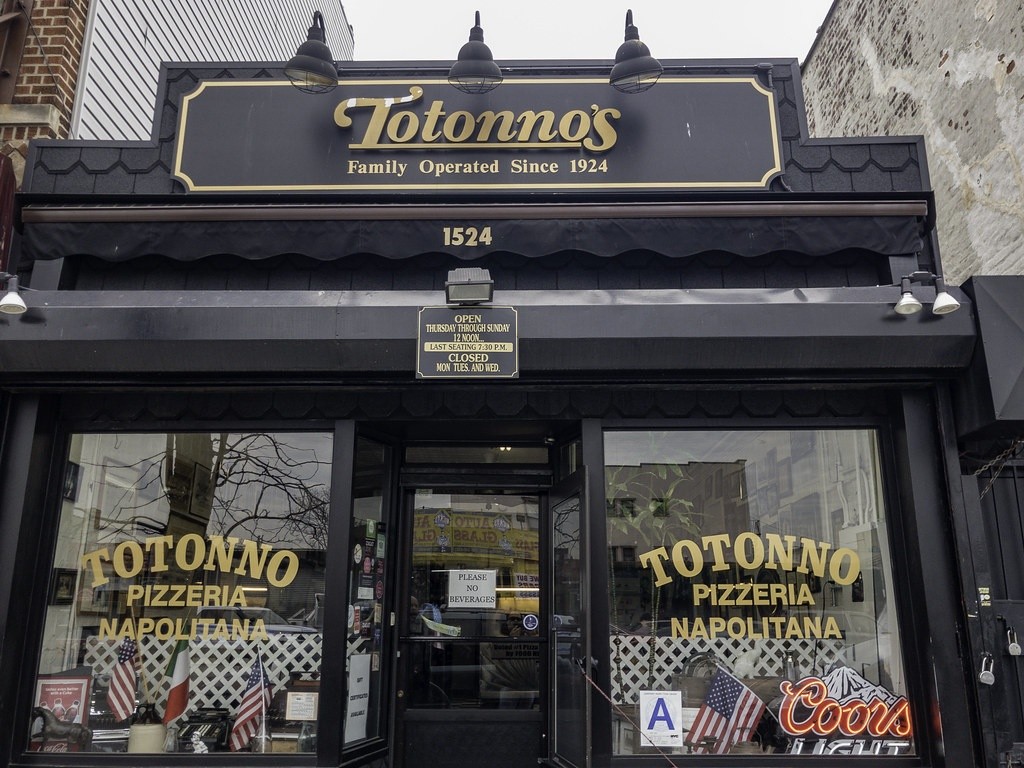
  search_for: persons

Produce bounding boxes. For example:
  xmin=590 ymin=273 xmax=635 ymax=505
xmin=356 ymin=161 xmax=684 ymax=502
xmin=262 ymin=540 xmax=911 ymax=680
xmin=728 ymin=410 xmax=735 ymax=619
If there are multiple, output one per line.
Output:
xmin=407 ymin=590 xmax=583 ymax=724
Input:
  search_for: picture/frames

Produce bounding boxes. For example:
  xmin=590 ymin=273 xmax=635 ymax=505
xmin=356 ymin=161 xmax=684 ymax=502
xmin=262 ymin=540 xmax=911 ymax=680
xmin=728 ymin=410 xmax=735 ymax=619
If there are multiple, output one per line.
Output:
xmin=606 ymin=430 xmax=863 ymax=630
xmin=48 ymin=456 xmax=231 ymax=676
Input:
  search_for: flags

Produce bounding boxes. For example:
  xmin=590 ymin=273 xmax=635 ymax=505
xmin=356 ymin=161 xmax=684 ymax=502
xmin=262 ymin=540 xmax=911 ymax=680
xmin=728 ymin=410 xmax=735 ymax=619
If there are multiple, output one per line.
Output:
xmin=684 ymin=668 xmax=765 ymax=756
xmin=228 ymin=650 xmax=272 ymax=753
xmin=161 ymin=620 xmax=190 ymax=731
xmin=105 ymin=617 xmax=138 ymax=725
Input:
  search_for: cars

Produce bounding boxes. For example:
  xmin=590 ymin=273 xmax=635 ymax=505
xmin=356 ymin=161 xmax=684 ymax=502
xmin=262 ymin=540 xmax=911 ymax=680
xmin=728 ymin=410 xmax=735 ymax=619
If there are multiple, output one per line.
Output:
xmin=197 ymin=606 xmax=318 ymax=634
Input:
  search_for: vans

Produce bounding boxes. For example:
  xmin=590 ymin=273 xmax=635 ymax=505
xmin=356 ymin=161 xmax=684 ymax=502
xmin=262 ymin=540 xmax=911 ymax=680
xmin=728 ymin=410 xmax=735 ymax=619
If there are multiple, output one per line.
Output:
xmin=789 ymin=611 xmax=891 ymax=645
xmin=496 ymin=588 xmax=539 ymax=614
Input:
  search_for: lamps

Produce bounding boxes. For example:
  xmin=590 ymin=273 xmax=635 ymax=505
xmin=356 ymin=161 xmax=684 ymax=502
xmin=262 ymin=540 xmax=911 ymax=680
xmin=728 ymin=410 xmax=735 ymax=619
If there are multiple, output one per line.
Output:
xmin=444 ymin=267 xmax=494 ymax=307
xmin=0 ymin=275 xmax=39 ymax=315
xmin=893 ymin=270 xmax=961 ymax=317
